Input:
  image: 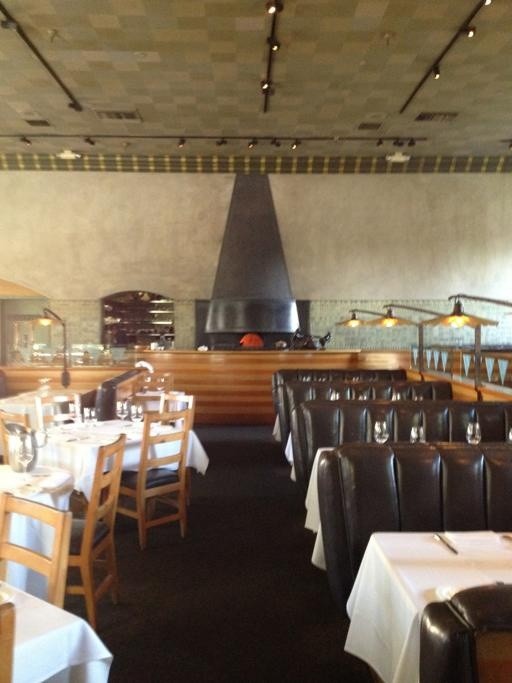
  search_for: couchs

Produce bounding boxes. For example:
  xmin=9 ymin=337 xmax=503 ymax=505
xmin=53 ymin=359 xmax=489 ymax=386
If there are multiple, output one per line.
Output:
xmin=420 ymin=585 xmax=511 ymax=682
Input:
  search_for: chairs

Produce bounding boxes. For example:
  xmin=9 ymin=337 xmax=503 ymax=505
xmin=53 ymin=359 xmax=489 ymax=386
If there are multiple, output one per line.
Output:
xmin=0 ymin=387 xmax=210 ymax=551
xmin=0 ymin=491 xmax=73 ymax=610
xmin=294 ymin=397 xmax=512 ymax=514
xmin=272 ymin=367 xmax=405 ymax=448
xmin=280 ymin=381 xmax=452 ymax=493
xmin=64 ymin=434 xmax=126 ymax=630
xmin=316 ymin=439 xmax=512 ymax=621
xmin=1 ymin=602 xmax=15 ymax=682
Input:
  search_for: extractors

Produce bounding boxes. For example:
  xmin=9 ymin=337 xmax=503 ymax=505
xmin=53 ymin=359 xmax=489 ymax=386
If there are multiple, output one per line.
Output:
xmin=203 ymin=174 xmax=301 ymax=334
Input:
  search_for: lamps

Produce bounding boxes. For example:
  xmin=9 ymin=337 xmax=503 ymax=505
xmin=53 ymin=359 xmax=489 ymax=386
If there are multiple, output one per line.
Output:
xmin=419 ymin=295 xmax=512 ymax=327
xmin=38 ymin=307 xmax=71 ymax=390
xmin=365 ymin=304 xmax=444 ymax=327
xmin=335 ymin=309 xmax=386 ymax=328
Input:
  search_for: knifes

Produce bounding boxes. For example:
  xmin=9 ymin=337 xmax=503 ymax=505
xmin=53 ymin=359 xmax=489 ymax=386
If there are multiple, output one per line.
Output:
xmin=436 ymin=532 xmax=459 ymax=554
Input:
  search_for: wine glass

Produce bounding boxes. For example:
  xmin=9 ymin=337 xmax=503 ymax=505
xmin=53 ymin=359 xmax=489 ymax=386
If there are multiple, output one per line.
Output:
xmin=409 ymin=426 xmax=427 ymax=444
xmin=83 ymin=407 xmax=100 ymax=436
xmin=372 ymin=420 xmax=389 ymax=444
xmin=38 ymin=378 xmax=50 ymax=397
xmin=466 ymin=422 xmax=482 ymax=446
xmin=16 ymin=433 xmax=34 ymax=486
xmin=114 ymin=400 xmax=129 ymax=427
xmin=130 ymin=404 xmax=144 ymax=431
